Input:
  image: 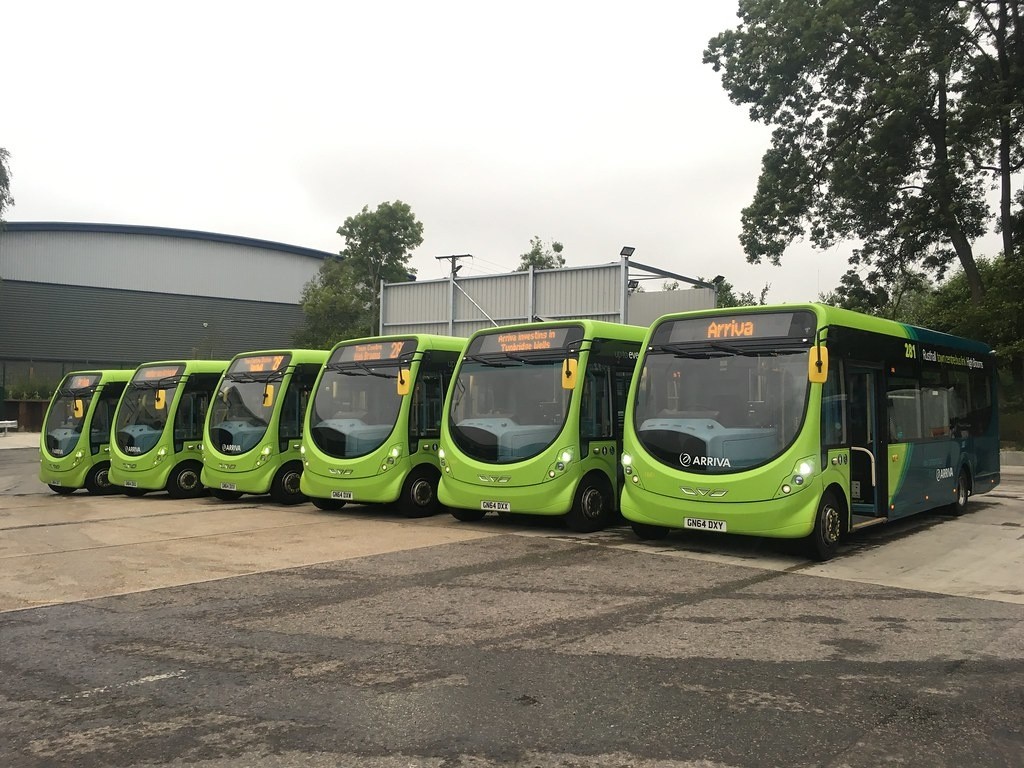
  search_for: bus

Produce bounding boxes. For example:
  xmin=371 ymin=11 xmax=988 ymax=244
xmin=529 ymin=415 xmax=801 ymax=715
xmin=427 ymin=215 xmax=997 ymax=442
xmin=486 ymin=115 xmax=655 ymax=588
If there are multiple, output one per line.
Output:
xmin=437 ymin=318 xmax=650 ymax=533
xmin=108 ymin=360 xmax=230 ymax=498
xmin=620 ymin=302 xmax=1000 ymax=562
xmin=38 ymin=370 xmax=137 ymax=495
xmin=200 ymin=349 xmax=331 ymax=507
xmin=301 ymin=334 xmax=470 ymax=519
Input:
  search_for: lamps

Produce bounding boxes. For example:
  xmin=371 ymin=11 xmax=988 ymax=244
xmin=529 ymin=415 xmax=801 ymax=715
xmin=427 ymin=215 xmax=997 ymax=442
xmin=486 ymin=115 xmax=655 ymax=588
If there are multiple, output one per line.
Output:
xmin=620 ymin=247 xmax=635 ymax=257
xmin=628 ymin=280 xmax=639 ymax=288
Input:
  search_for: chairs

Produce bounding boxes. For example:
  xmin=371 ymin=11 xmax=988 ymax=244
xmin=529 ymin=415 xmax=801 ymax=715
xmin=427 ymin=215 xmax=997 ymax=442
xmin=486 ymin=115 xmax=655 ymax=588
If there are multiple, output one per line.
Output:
xmin=361 ymin=399 xmax=386 ymax=425
xmin=710 ymin=398 xmax=737 ymax=427
xmin=91 ymin=402 xmax=106 ymax=433
